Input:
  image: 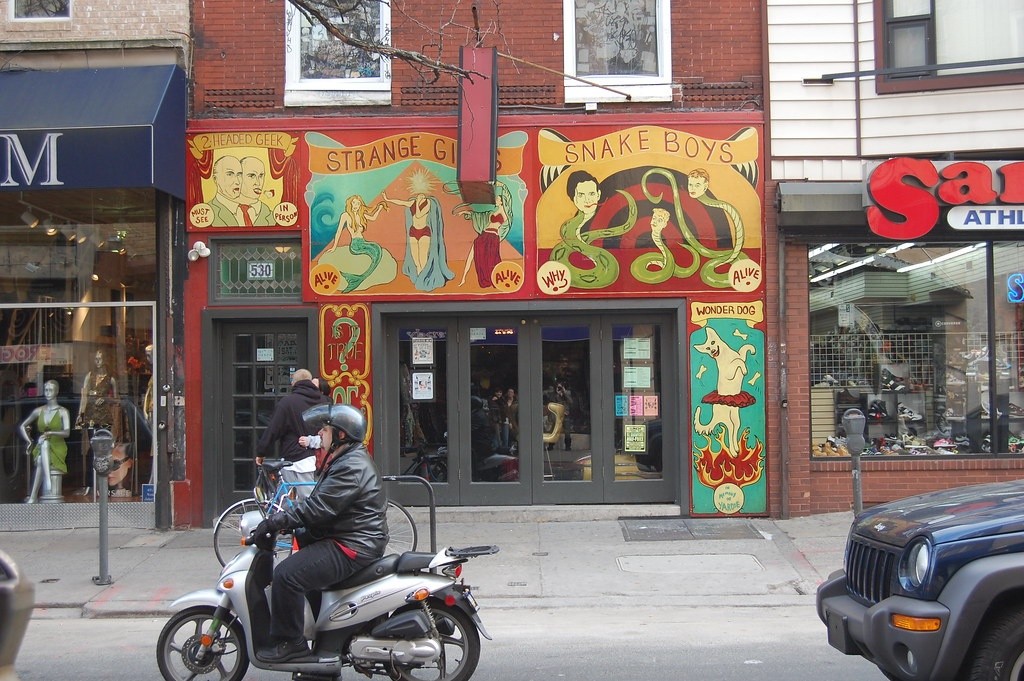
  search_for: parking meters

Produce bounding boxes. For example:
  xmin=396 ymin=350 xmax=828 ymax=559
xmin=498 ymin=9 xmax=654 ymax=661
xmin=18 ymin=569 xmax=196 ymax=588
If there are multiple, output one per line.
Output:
xmin=839 ymin=407 xmax=868 ymax=518
xmin=88 ymin=430 xmax=116 ymax=587
xmin=0 ymin=552 xmax=36 ymax=681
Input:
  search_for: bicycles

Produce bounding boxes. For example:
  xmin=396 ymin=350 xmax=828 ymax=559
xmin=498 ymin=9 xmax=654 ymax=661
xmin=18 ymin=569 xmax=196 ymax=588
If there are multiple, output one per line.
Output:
xmin=212 ymin=456 xmax=418 ymax=568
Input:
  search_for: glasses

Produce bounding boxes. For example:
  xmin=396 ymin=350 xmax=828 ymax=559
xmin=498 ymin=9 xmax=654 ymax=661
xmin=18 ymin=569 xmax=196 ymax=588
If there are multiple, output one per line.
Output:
xmin=110 ymin=455 xmax=131 ymax=471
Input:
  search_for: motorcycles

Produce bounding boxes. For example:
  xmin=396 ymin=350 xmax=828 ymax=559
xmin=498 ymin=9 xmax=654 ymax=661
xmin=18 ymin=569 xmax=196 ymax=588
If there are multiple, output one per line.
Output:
xmin=155 ymin=488 xmax=501 ymax=681
xmin=412 ymin=431 xmax=518 ymax=483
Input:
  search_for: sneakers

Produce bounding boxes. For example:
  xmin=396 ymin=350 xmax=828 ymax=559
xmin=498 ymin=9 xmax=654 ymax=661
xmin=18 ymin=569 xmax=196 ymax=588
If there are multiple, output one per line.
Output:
xmin=256 ymin=636 xmax=311 ymax=663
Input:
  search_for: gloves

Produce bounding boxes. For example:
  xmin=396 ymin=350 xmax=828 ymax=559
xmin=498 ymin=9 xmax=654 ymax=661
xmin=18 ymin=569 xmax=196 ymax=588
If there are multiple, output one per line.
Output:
xmin=254 ymin=518 xmax=277 ymax=552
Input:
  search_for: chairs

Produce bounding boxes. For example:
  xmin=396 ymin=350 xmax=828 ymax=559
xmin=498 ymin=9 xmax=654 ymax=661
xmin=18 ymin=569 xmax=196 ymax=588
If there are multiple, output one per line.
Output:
xmin=542 ymin=403 xmax=565 ymax=478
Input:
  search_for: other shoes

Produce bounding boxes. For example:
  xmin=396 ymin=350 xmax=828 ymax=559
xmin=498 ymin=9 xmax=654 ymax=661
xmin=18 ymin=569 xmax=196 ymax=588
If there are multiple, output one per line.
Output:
xmin=811 ymin=345 xmax=1024 ymax=457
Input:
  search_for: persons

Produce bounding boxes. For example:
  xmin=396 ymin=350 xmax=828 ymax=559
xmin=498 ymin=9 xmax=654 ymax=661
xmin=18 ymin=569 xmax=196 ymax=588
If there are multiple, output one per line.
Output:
xmin=544 ymin=377 xmax=572 ymax=452
xmin=255 ymin=404 xmax=390 ymax=663
xmin=19 ymin=380 xmax=71 ymax=503
xmin=471 ymin=387 xmax=519 ymax=462
xmin=143 ymin=345 xmax=153 ymax=484
xmin=108 ymin=443 xmax=133 ymax=490
xmin=255 ymin=369 xmax=334 ymax=534
xmin=76 ymin=345 xmax=119 ymax=496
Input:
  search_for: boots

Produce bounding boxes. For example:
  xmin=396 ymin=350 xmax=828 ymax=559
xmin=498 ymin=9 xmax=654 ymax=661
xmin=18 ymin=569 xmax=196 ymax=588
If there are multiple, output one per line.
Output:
xmin=547 ymin=443 xmax=555 ymax=451
xmin=565 ymin=437 xmax=572 ymax=451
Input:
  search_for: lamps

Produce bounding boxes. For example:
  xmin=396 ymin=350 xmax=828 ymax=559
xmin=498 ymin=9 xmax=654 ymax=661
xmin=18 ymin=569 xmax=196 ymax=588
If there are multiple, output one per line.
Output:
xmin=90 ymin=271 xmax=112 ymax=285
xmin=188 ymin=241 xmax=214 ymax=261
xmin=17 ymin=202 xmax=130 ymax=255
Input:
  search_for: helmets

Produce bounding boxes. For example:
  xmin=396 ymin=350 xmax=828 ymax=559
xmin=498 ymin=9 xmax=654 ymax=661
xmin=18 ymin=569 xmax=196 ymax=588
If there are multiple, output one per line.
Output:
xmin=471 ymin=396 xmax=484 ymax=410
xmin=320 ymin=403 xmax=365 ymax=442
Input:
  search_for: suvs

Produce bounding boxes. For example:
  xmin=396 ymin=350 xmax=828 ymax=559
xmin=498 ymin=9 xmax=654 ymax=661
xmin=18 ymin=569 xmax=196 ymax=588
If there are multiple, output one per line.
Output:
xmin=619 ymin=417 xmax=664 ymax=473
xmin=814 ymin=480 xmax=1024 ymax=681
xmin=0 ymin=394 xmax=153 ymax=499
